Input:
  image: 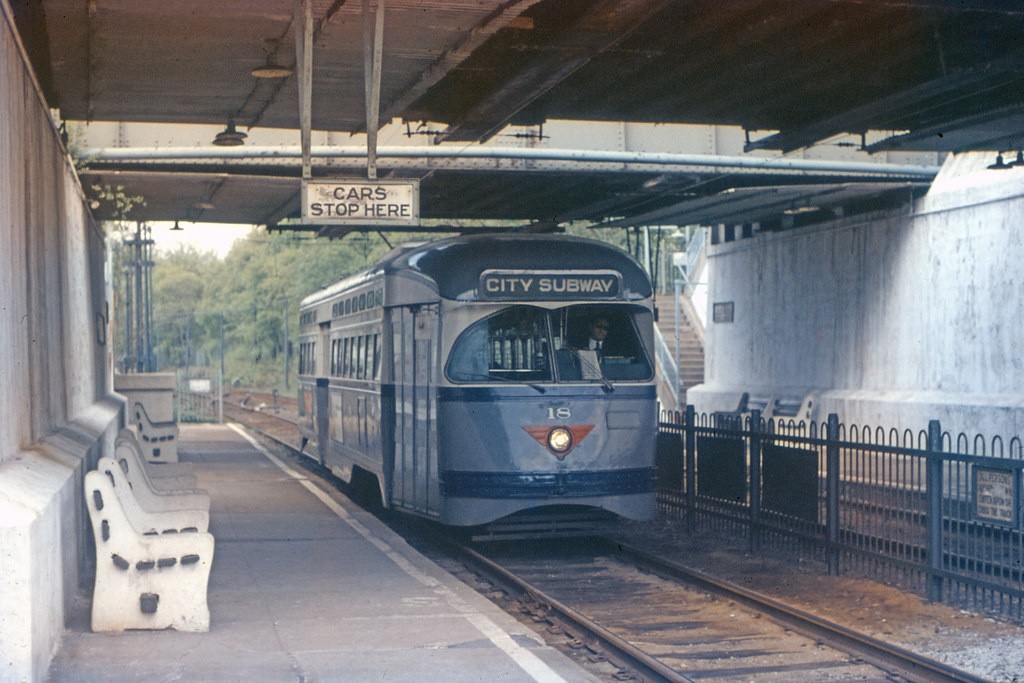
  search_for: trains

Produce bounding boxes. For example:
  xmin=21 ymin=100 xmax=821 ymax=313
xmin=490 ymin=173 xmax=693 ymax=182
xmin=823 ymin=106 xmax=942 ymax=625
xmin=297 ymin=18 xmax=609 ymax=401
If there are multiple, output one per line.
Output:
xmin=297 ymin=230 xmax=661 ymax=543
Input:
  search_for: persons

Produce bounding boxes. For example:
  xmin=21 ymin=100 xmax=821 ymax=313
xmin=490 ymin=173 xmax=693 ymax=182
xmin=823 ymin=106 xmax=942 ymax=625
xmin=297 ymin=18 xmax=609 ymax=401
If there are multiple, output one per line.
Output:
xmin=578 ymin=314 xmax=617 ymax=362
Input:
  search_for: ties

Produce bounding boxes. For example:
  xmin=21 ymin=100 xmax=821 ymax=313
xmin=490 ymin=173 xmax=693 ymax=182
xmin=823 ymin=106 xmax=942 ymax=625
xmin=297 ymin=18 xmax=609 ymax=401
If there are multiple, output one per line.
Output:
xmin=594 ymin=343 xmax=601 ymax=362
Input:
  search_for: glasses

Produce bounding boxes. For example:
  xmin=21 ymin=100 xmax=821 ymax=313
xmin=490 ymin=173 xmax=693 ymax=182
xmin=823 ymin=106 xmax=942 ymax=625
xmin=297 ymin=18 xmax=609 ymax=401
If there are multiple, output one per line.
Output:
xmin=595 ymin=325 xmax=608 ymax=330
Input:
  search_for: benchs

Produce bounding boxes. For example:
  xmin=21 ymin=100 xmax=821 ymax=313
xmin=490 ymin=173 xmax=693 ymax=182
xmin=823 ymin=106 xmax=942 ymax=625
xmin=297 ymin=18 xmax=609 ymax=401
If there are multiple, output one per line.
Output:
xmin=83 ymin=430 xmax=214 ymax=633
xmin=133 ymin=402 xmax=181 ymax=463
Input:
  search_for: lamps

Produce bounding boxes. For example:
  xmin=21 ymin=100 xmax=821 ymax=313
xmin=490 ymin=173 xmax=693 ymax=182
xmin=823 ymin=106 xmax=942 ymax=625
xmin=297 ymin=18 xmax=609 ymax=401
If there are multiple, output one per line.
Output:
xmin=169 ymin=214 xmax=185 ymax=231
xmin=189 ymin=181 xmax=217 ymax=210
xmin=216 ymin=116 xmax=248 ymax=139
xmin=213 ymin=138 xmax=244 ymax=146
xmin=986 ymin=150 xmax=1012 ymax=169
xmin=1008 ymin=150 xmax=1024 ymax=166
xmin=250 ymin=54 xmax=293 ymax=78
